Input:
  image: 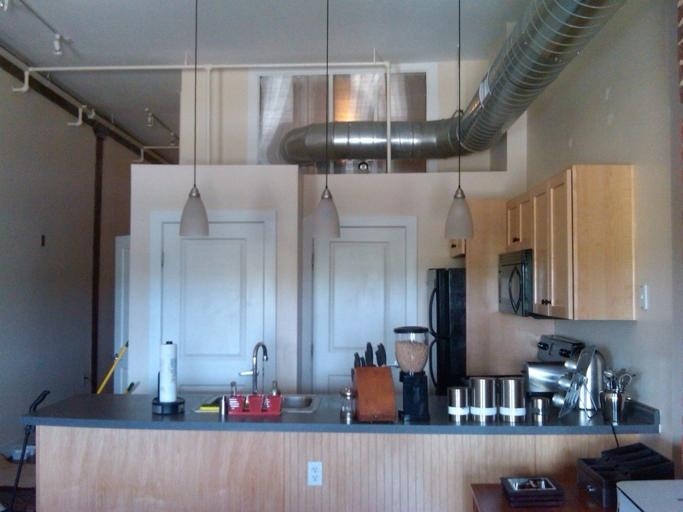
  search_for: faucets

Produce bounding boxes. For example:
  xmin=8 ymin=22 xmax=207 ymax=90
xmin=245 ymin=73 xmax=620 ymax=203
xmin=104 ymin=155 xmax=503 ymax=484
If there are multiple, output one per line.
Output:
xmin=251 ymin=339 xmax=269 ymax=394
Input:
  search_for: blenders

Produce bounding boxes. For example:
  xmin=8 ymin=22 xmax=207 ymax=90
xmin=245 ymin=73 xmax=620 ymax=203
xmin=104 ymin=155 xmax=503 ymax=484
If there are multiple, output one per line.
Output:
xmin=393 ymin=324 xmax=435 ymax=424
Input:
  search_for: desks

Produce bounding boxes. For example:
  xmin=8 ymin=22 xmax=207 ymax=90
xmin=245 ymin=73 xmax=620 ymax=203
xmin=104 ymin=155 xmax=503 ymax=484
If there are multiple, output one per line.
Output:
xmin=469 ymin=475 xmax=615 ymax=511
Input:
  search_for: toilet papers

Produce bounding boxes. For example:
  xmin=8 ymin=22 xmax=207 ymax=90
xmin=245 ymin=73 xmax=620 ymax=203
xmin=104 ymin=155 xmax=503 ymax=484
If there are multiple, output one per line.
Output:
xmin=159 ymin=343 xmax=180 ymax=404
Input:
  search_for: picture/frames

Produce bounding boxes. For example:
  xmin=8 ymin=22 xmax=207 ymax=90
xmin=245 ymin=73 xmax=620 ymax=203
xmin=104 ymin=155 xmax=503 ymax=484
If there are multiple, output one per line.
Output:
xmin=498 ymin=474 xmax=566 ymax=508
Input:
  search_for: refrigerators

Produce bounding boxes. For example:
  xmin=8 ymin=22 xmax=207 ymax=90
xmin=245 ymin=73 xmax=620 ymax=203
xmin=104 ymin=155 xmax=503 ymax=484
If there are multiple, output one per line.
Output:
xmin=426 ymin=267 xmax=467 ymax=396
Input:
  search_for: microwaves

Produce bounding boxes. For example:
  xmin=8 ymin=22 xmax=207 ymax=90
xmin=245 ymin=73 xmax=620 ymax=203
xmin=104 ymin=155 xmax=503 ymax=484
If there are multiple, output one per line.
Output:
xmin=497 ymin=249 xmax=534 ymax=318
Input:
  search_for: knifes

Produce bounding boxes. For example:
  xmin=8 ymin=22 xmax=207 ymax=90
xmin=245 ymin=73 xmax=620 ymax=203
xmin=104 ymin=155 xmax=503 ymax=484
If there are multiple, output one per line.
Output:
xmin=355 ymin=341 xmax=388 ymax=367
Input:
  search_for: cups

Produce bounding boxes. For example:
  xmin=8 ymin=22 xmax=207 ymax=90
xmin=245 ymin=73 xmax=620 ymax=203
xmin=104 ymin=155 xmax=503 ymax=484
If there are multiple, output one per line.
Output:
xmin=446 ymin=376 xmax=551 ymax=424
xmin=600 ymin=389 xmax=631 ymax=424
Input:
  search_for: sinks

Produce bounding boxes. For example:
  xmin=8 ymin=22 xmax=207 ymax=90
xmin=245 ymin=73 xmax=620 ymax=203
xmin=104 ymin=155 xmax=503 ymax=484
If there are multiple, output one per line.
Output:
xmin=200 ymin=393 xmax=319 ymax=414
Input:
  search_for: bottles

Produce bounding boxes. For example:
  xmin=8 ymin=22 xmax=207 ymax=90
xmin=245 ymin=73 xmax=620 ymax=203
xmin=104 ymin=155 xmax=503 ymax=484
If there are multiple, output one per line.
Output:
xmin=340 ymin=386 xmax=358 ymax=424
xmin=219 ymin=394 xmax=229 ymax=417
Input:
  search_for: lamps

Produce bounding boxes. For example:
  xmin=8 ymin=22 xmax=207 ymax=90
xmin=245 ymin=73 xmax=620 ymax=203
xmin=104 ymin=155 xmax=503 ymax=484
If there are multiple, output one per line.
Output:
xmin=177 ymin=0 xmax=210 ymax=239
xmin=307 ymin=0 xmax=340 ymax=241
xmin=442 ymin=0 xmax=474 ymax=240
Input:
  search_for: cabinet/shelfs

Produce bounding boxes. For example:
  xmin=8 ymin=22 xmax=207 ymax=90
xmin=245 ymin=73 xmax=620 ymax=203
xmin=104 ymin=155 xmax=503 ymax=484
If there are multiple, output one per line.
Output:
xmin=534 ymin=167 xmax=573 ymax=317
xmin=505 ymin=191 xmax=532 ymax=243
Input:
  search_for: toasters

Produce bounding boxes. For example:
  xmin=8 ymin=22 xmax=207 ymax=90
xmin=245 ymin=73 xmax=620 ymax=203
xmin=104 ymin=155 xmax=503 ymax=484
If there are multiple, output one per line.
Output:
xmin=524 ymin=360 xmax=570 ymax=398
